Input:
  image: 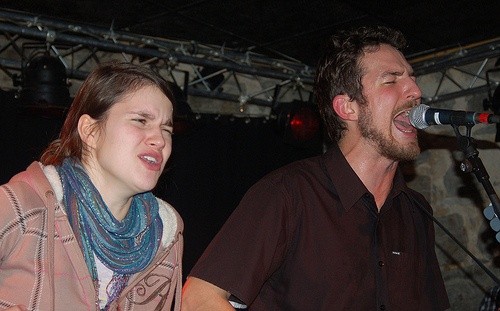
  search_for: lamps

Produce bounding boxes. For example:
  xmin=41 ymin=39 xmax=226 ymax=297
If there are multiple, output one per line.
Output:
xmin=150 ymin=60 xmax=197 ymax=134
xmin=269 ymin=84 xmax=322 ymax=149
xmin=14 ymin=41 xmax=73 ymax=109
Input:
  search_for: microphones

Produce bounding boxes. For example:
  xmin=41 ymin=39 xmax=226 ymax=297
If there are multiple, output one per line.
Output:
xmin=409 ymin=104 xmax=500 ymax=130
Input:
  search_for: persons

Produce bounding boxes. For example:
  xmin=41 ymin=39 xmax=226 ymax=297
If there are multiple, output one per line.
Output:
xmin=181 ymin=25 xmax=451 ymax=311
xmin=0 ymin=64 xmax=185 ymax=311
